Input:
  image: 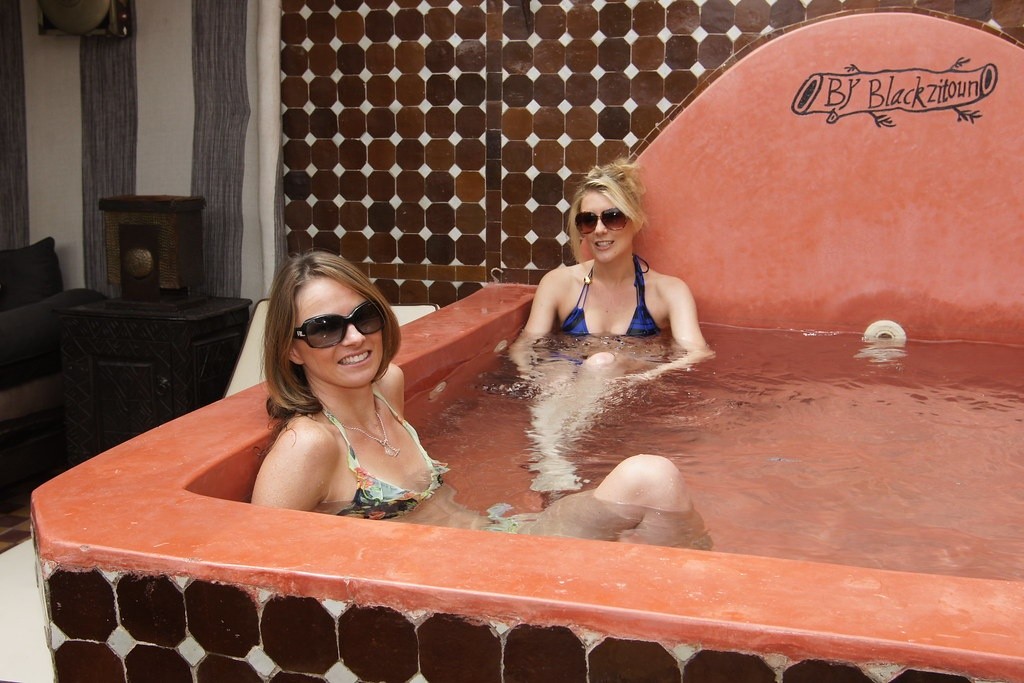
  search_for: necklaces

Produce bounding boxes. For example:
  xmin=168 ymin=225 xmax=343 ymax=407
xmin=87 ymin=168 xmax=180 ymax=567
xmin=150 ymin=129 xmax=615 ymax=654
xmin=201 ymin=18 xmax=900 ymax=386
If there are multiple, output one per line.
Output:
xmin=342 ymin=410 xmax=401 ymax=458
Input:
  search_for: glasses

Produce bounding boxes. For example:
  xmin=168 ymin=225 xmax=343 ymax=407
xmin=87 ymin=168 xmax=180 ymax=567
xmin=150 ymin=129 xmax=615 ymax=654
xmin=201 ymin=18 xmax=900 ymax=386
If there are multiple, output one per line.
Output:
xmin=575 ymin=207 xmax=640 ymax=238
xmin=292 ymin=300 xmax=385 ymax=349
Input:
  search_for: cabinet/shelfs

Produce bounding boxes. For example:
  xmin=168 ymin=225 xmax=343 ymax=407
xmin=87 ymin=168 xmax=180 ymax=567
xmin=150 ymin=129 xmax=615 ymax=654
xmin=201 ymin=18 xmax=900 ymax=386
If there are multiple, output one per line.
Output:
xmin=55 ymin=296 xmax=251 ymax=470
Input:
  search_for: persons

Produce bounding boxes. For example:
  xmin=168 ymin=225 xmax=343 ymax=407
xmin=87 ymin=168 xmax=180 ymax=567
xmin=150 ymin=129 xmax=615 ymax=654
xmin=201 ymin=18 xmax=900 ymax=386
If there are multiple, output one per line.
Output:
xmin=250 ymin=251 xmax=713 ymax=552
xmin=507 ymin=160 xmax=715 ymax=509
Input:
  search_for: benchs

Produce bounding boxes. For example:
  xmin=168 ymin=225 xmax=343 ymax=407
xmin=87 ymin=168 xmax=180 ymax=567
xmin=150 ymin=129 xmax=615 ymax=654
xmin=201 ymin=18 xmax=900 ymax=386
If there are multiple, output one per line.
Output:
xmin=0 ymin=237 xmax=108 ymax=495
